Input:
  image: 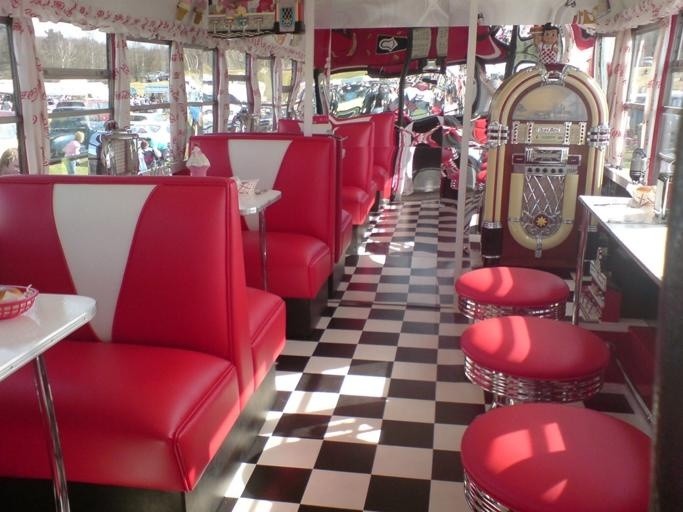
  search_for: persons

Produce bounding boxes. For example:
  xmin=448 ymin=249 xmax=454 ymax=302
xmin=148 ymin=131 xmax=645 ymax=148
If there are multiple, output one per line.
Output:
xmin=126 ymin=90 xmax=161 ymax=114
xmin=63 ymin=130 xmax=85 ymax=174
xmin=140 ymin=140 xmax=158 ymax=172
xmin=0 ymin=91 xmax=17 ymax=113
xmin=0 ymin=144 xmax=21 ymax=175
xmin=530 ymin=23 xmax=560 ymax=65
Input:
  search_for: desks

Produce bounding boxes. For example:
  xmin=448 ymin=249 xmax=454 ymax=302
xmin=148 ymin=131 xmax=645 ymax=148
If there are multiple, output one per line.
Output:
xmin=572 ymin=195 xmax=668 ymax=424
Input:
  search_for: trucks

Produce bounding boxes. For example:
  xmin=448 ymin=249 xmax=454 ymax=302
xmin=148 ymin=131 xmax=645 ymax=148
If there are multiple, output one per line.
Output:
xmin=48 ymin=98 xmax=109 ymax=158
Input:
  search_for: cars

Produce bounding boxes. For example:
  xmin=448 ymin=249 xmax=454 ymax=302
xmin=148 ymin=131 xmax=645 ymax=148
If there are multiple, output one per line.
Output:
xmin=628 ymin=91 xmax=682 ymax=148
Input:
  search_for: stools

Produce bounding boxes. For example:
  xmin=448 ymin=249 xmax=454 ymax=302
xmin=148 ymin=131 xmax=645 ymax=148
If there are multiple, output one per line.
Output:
xmin=460 ymin=402 xmax=652 ymax=512
xmin=461 ymin=315 xmax=612 ymax=405
xmin=455 ymin=267 xmax=570 ymax=323
